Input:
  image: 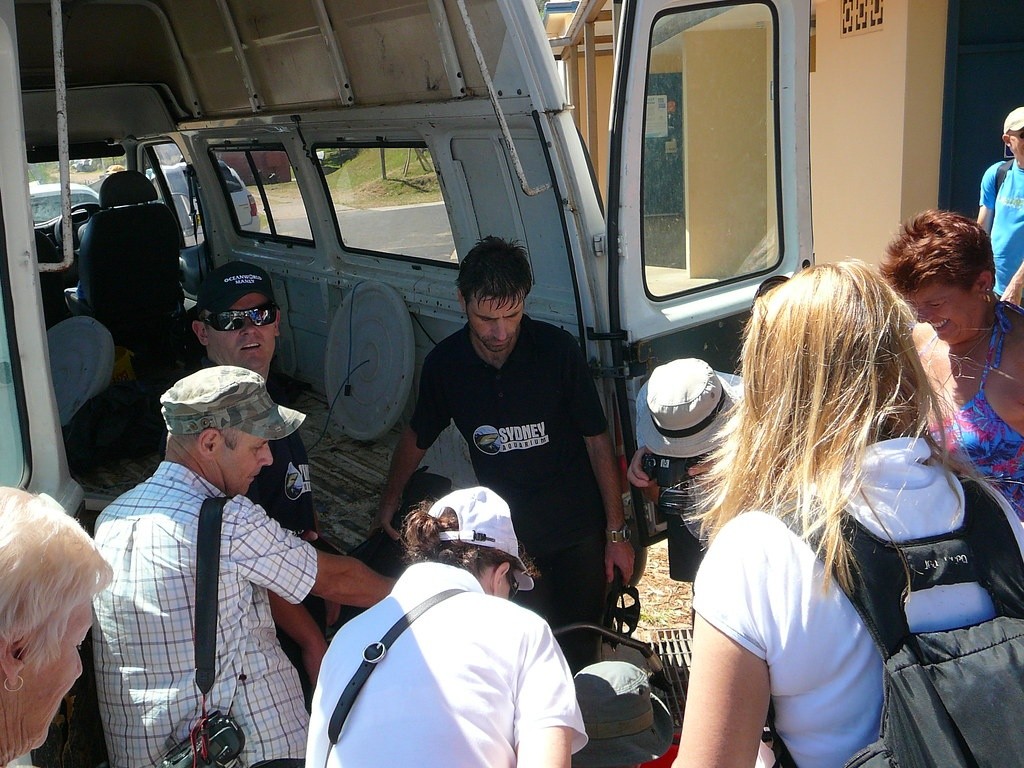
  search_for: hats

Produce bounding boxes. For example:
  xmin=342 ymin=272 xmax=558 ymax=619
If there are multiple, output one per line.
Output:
xmin=160 ymin=365 xmax=307 ymax=442
xmin=636 ymin=357 xmax=747 ymax=459
xmin=1002 ymin=106 xmax=1024 ymax=134
xmin=571 ymin=661 xmax=674 ymax=767
xmin=424 ymin=486 xmax=534 ymax=591
xmin=199 ymin=262 xmax=278 ymax=319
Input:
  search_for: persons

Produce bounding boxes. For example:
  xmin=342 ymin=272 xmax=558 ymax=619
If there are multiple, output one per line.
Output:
xmin=0 ymin=486 xmax=113 ymax=768
xmin=976 ymin=106 xmax=1024 ymax=306
xmin=90 ymin=210 xmax=1024 ymax=768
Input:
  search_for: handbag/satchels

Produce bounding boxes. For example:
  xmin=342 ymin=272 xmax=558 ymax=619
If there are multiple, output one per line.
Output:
xmin=251 ymin=758 xmax=306 ymax=768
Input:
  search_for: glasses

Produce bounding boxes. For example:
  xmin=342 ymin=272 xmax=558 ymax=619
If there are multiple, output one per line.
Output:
xmin=203 ymin=303 xmax=278 ymax=333
xmin=1010 ymin=132 xmax=1024 ymax=138
xmin=493 ymin=555 xmax=519 ymax=599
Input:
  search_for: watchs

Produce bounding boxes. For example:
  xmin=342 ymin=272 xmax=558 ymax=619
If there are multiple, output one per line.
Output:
xmin=607 ymin=524 xmax=630 ymax=543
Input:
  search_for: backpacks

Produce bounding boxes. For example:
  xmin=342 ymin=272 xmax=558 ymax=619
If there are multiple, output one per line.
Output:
xmin=830 ymin=467 xmax=1024 ymax=766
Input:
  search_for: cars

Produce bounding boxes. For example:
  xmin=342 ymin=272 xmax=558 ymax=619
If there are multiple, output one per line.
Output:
xmin=29 ymin=184 xmax=99 ymax=224
xmin=85 ymin=161 xmax=260 ymax=247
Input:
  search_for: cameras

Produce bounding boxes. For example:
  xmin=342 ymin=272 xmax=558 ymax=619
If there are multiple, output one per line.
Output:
xmin=155 ymin=711 xmax=246 ymax=768
xmin=640 ymin=453 xmax=705 ymax=514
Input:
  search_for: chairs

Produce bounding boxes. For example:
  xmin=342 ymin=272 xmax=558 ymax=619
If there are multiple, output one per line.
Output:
xmin=32 ymin=169 xmax=186 ymax=344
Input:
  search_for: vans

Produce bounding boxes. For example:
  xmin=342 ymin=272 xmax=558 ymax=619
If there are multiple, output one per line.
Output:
xmin=3 ymin=0 xmax=814 ymax=768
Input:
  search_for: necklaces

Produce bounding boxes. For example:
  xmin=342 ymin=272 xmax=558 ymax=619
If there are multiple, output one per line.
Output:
xmin=924 ymin=331 xmax=991 ymax=403
